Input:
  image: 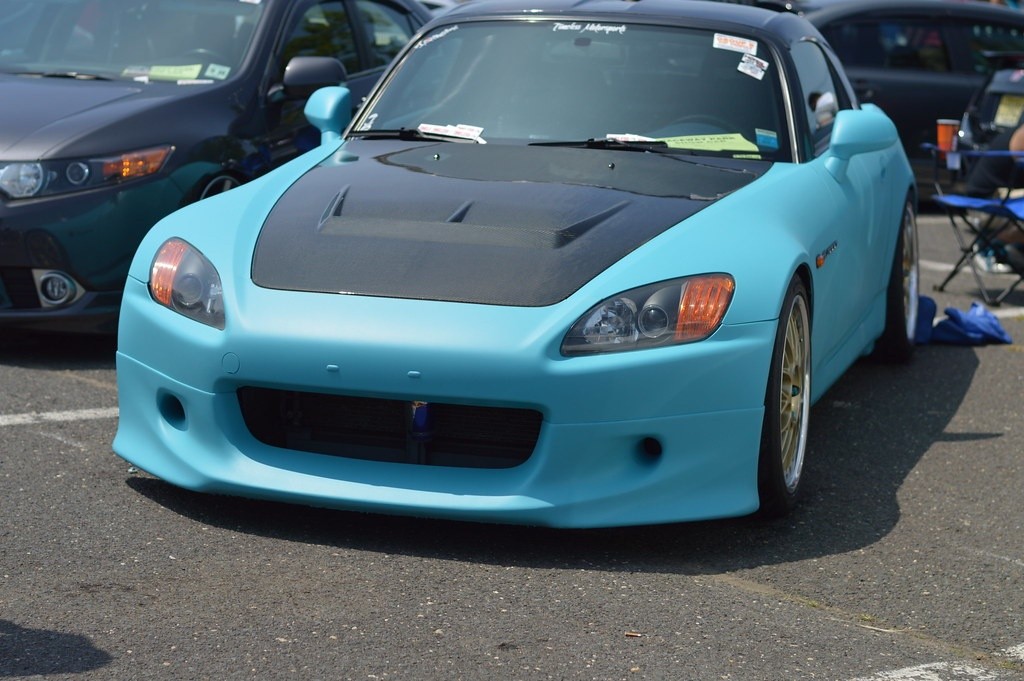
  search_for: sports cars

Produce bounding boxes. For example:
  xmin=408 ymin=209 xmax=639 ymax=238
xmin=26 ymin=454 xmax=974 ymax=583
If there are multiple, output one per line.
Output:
xmin=113 ymin=0 xmax=925 ymax=542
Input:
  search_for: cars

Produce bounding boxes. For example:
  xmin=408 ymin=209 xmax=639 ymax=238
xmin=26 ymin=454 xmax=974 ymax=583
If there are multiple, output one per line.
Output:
xmin=0 ymin=0 xmax=451 ymax=354
xmin=701 ymin=0 xmax=1024 ymax=214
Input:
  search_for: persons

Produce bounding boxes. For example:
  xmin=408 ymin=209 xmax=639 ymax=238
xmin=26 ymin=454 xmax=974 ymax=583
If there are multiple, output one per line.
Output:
xmin=963 ymin=119 xmax=1024 ymax=275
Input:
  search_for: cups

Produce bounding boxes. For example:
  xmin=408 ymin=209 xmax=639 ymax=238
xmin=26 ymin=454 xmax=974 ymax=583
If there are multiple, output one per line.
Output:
xmin=935 ymin=118 xmax=960 ymax=160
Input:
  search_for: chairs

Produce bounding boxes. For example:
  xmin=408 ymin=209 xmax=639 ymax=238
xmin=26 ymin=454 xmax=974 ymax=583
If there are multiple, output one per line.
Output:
xmin=124 ymin=3 xmax=892 ymax=159
xmin=924 ymin=140 xmax=1023 ymax=305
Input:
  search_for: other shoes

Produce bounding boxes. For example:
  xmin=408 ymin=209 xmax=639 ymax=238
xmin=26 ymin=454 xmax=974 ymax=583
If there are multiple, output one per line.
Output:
xmin=971 ymin=245 xmax=1013 ymax=272
